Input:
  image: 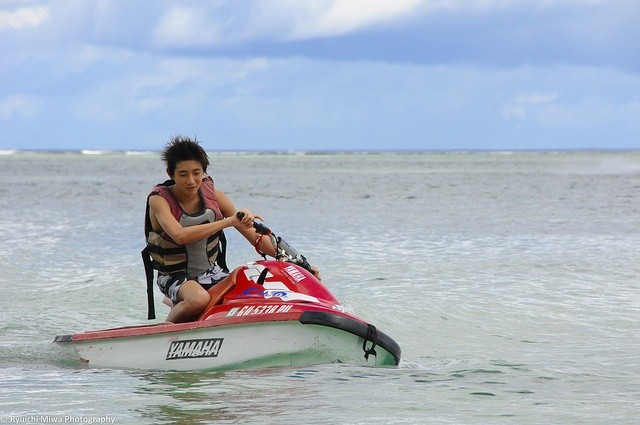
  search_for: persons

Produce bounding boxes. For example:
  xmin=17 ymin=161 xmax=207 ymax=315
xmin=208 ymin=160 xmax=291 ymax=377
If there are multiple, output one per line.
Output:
xmin=145 ymin=133 xmax=322 ymax=322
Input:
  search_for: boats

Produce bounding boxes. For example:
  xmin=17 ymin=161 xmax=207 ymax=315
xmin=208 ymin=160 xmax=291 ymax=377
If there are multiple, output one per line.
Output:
xmin=54 ymin=212 xmax=401 ymax=371
